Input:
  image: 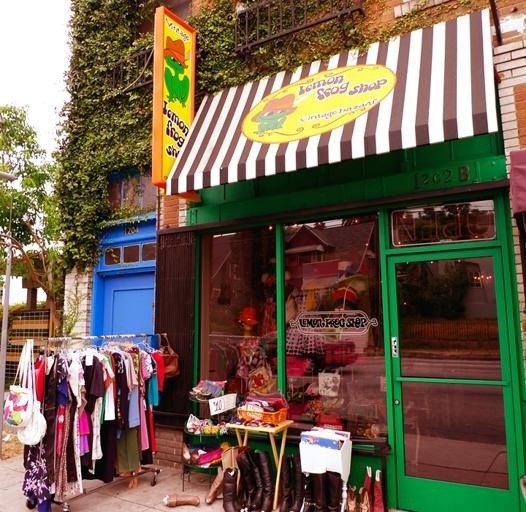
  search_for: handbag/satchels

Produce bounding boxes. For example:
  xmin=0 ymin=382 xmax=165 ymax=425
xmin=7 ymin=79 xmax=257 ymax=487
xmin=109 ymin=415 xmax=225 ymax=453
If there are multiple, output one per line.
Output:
xmin=318 ymin=368 xmax=340 ymax=397
xmin=17 ymin=410 xmax=47 ymax=446
xmin=3 ymin=339 xmax=33 ymax=427
xmin=159 ymin=335 xmax=179 ymax=377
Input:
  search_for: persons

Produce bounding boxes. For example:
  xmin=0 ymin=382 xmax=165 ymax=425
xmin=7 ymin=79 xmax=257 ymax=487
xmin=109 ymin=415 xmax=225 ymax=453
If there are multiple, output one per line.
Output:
xmin=234 ymin=306 xmax=271 ymax=395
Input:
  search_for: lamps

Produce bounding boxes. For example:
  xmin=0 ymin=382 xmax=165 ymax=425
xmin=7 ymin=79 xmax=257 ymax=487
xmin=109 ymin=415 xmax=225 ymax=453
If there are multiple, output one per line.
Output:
xmin=471 ymin=270 xmax=492 ymax=289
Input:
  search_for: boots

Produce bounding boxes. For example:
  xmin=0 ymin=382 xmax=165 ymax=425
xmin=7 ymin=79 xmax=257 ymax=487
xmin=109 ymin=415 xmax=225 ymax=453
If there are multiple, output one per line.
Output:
xmin=244 ymin=453 xmax=264 ymax=512
xmin=223 ymin=468 xmax=237 ymax=512
xmin=205 ymin=467 xmax=223 ymax=504
xmin=359 ymin=487 xmax=370 ymax=512
xmin=168 ymin=494 xmax=200 ymax=507
xmin=280 ymin=456 xmax=292 ymax=512
xmin=326 ymin=471 xmax=341 ymax=512
xmin=256 ymin=452 xmax=272 ymax=512
xmin=374 ymin=470 xmax=385 ymax=512
xmin=346 ymin=485 xmax=358 ymax=512
xmin=303 ymin=472 xmax=315 ymax=512
xmin=364 ymin=466 xmax=373 ymax=512
xmin=310 ymin=473 xmax=326 ymax=512
xmin=287 ymin=456 xmax=303 ymax=512
xmin=236 ymin=454 xmax=256 ymax=512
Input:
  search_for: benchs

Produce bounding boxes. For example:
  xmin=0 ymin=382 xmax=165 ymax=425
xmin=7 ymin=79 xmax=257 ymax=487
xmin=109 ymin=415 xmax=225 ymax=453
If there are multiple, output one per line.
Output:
xmin=398 ymin=317 xmax=501 ymax=361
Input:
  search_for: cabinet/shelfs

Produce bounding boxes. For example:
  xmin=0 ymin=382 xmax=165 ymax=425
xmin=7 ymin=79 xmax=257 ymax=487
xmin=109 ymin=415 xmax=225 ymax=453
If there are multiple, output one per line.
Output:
xmin=181 ymin=379 xmax=228 ymax=490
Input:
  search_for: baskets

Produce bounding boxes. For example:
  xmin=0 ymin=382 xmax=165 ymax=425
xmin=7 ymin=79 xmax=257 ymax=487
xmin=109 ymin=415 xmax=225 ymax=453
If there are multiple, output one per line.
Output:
xmin=239 ymin=408 xmax=287 ymax=424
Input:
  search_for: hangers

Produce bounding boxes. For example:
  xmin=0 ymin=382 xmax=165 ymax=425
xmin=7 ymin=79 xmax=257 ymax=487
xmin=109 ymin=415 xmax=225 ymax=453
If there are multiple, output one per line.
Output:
xmin=44 ymin=333 xmax=159 ymax=355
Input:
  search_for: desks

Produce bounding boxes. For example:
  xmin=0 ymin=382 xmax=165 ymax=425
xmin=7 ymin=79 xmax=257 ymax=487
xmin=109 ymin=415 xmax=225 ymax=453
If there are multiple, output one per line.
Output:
xmin=225 ymin=418 xmax=295 ymax=512
